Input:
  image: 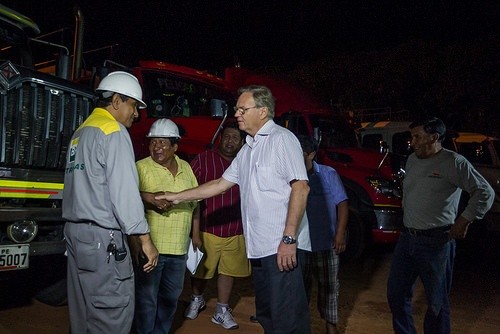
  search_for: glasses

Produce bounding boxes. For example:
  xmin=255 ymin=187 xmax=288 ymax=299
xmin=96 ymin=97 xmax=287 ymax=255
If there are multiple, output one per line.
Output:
xmin=234 ymin=106 xmax=260 ymax=114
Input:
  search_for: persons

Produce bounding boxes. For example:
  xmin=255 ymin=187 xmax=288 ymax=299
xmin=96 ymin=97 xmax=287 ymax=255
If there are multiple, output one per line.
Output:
xmin=185 ymin=122 xmax=251 ymax=330
xmin=386 ymin=116 xmax=496 ymax=334
xmin=295 ymin=135 xmax=348 ymax=334
xmin=155 ymin=86 xmax=310 ymax=334
xmin=136 ymin=118 xmax=202 ymax=334
xmin=61 ymin=71 xmax=174 ymax=334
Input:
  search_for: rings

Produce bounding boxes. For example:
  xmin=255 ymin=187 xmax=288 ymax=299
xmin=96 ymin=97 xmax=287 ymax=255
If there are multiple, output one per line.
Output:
xmin=164 ymin=204 xmax=166 ymax=207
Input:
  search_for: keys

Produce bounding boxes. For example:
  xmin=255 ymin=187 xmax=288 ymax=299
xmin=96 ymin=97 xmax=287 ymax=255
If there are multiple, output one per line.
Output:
xmin=105 ymin=242 xmax=117 ymax=264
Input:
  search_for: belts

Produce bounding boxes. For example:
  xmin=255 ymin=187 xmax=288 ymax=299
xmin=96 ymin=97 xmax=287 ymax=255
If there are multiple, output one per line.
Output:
xmin=403 ymin=225 xmax=452 ymax=236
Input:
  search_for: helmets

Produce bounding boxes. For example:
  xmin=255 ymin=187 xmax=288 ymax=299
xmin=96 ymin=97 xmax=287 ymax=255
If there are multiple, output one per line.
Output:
xmin=147 ymin=118 xmax=180 ymax=137
xmin=96 ymin=71 xmax=147 ymax=110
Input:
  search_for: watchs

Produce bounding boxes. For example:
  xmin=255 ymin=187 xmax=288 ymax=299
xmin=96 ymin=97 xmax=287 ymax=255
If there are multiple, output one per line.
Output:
xmin=281 ymin=235 xmax=297 ymax=245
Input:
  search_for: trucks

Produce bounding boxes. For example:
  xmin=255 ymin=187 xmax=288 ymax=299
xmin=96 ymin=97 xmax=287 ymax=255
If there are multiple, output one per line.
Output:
xmin=97 ymin=59 xmax=405 ymax=268
xmin=0 ymin=3 xmax=99 ymax=307
xmin=352 ymin=119 xmax=500 ymax=216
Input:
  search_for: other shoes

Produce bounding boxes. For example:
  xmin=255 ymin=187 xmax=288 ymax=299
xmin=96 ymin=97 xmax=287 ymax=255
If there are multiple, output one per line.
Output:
xmin=251 ymin=314 xmax=259 ymax=323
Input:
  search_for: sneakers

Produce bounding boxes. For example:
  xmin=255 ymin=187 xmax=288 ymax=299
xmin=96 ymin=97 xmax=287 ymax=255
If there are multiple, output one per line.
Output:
xmin=183 ymin=295 xmax=206 ymax=320
xmin=211 ymin=305 xmax=238 ymax=330
